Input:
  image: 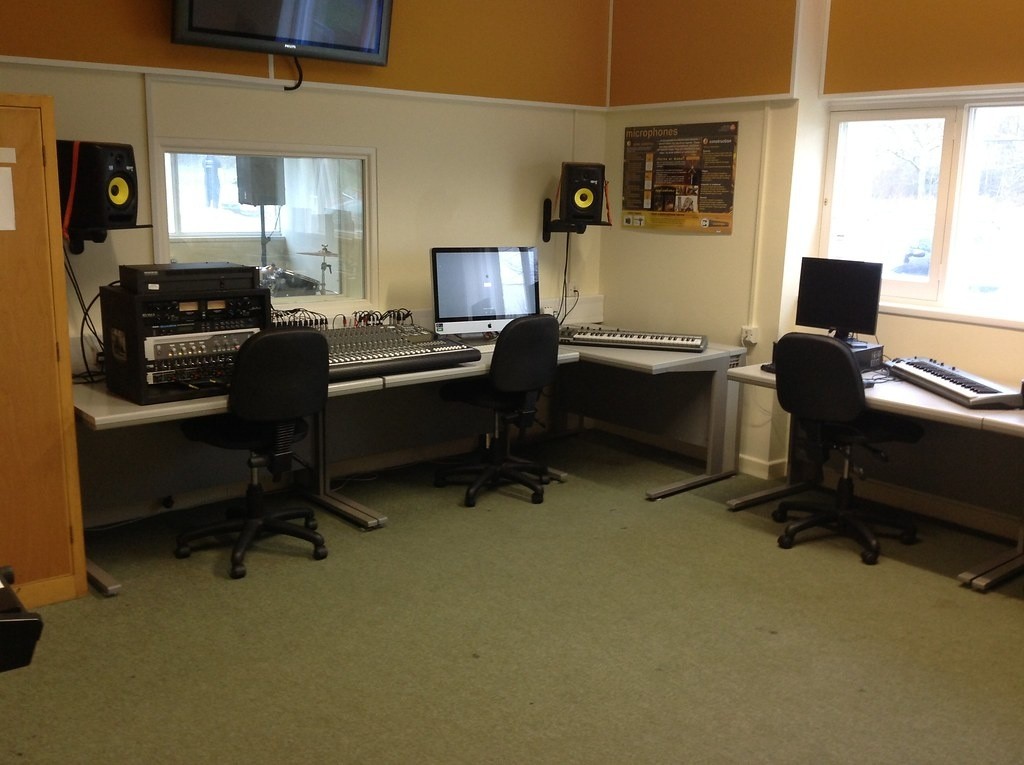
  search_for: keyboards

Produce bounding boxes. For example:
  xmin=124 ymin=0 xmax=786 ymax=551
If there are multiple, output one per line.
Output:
xmin=761 ymin=362 xmax=775 ymax=375
xmin=474 ymin=344 xmax=496 ymax=353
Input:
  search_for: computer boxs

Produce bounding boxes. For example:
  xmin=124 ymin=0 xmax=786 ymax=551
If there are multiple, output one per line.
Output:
xmin=851 ymin=342 xmax=885 ymax=375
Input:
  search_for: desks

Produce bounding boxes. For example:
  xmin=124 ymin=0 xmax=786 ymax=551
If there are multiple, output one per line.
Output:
xmin=559 ymin=334 xmax=747 ymax=498
xmin=725 ymin=360 xmax=1024 ymax=590
xmin=71 ymin=340 xmax=384 ymax=595
xmin=323 ymin=344 xmax=581 ymax=525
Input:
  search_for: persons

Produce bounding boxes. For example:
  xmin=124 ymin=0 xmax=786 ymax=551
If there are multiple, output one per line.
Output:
xmin=201 ymin=155 xmax=222 ymax=208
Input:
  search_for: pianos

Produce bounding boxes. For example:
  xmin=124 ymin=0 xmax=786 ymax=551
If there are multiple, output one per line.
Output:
xmin=883 ymin=354 xmax=1024 ymax=411
xmin=557 ymin=327 xmax=708 ymax=354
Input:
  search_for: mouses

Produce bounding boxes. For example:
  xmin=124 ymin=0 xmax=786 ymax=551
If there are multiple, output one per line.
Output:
xmin=862 ymin=379 xmax=874 ymax=389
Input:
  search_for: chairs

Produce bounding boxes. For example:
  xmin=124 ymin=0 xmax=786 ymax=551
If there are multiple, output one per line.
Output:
xmin=773 ymin=332 xmax=916 ymax=564
xmin=171 ymin=329 xmax=330 ymax=580
xmin=442 ymin=314 xmax=560 ymax=508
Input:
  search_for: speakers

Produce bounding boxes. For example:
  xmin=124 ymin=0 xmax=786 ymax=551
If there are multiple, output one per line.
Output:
xmin=236 ymin=154 xmax=286 ymax=206
xmin=55 ymin=139 xmax=138 ymax=229
xmin=559 ymin=161 xmax=605 ymax=223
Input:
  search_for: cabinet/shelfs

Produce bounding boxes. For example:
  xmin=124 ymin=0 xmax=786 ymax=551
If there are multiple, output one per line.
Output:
xmin=1 ymin=0 xmax=1024 ymax=607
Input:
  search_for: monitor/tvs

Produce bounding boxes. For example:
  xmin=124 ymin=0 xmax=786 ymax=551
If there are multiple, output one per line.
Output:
xmin=433 ymin=246 xmax=540 ymax=347
xmin=795 ymin=257 xmax=882 ymax=348
xmin=171 ymin=0 xmax=392 ymax=68
